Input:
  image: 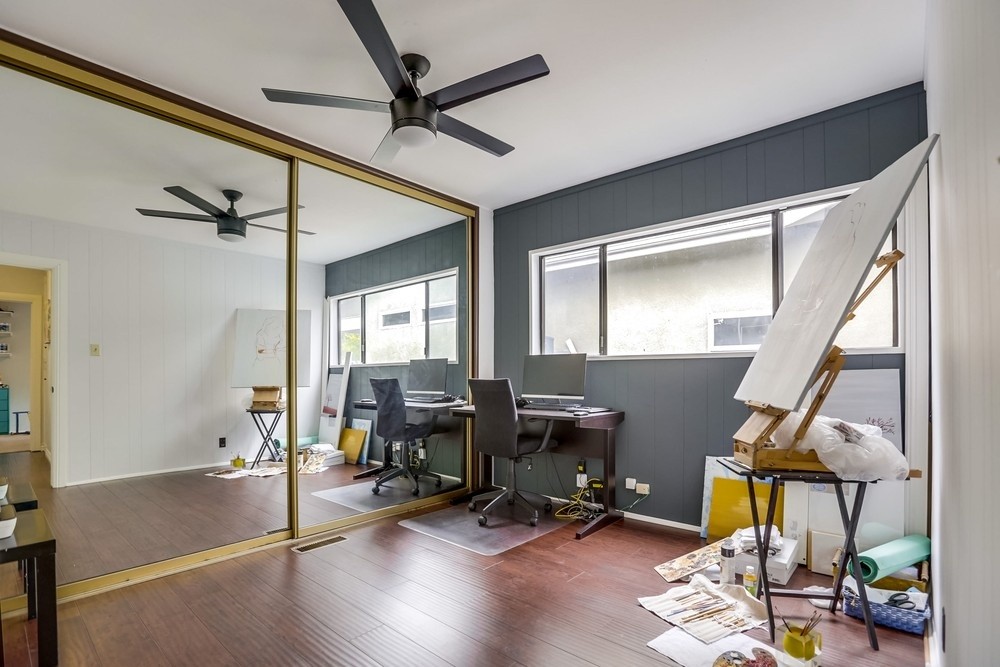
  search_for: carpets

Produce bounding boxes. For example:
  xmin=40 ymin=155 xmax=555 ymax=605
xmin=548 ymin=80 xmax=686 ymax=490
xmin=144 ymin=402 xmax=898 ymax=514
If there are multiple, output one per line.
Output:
xmin=310 ymin=470 xmax=458 ymax=512
xmin=397 ymin=501 xmax=577 ymax=558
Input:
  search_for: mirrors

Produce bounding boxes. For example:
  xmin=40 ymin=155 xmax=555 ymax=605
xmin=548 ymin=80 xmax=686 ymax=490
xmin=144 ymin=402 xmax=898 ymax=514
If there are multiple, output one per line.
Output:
xmin=0 ymin=25 xmax=289 ymax=608
xmin=291 ymin=151 xmax=475 ymax=535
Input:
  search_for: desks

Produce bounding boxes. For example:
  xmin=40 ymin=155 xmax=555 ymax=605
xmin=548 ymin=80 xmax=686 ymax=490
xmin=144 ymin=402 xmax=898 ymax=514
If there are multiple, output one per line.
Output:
xmin=448 ymin=402 xmax=624 ymax=541
xmin=718 ymin=457 xmax=923 ymax=656
xmin=242 ymin=406 xmax=286 ymax=469
xmin=0 ymin=471 xmax=37 ymax=596
xmin=0 ymin=509 xmax=60 ymax=667
xmin=350 ymin=397 xmax=467 ymax=490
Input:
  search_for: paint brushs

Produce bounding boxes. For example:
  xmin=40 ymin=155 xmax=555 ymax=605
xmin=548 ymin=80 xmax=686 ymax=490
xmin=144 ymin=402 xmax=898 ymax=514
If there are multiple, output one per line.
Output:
xmin=652 ymin=590 xmax=752 ymax=630
xmin=723 ymin=458 xmax=756 ymax=474
xmin=775 ymin=606 xmax=792 ymax=633
xmin=231 ymin=450 xmax=240 ymax=459
xmin=800 ymin=610 xmax=823 ymax=636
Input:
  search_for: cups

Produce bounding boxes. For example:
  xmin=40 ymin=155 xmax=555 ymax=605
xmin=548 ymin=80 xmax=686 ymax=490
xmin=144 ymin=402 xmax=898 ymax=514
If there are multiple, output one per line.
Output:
xmin=231 ymin=459 xmax=245 ymax=467
xmin=784 ymin=627 xmax=821 ymax=661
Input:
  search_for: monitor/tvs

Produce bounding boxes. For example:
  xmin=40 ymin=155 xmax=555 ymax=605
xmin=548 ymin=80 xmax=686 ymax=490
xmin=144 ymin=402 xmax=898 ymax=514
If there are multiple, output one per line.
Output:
xmin=407 ymin=359 xmax=447 ymax=400
xmin=520 ymin=354 xmax=588 ymax=407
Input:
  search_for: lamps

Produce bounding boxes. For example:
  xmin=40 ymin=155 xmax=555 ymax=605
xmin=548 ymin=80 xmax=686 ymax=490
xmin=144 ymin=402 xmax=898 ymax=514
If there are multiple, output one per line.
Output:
xmin=213 ymin=227 xmax=247 ymax=244
xmin=390 ymin=119 xmax=438 ymax=150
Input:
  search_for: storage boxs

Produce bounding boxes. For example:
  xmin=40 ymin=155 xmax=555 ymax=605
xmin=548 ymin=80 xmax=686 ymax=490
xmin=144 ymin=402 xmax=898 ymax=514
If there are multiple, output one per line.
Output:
xmin=301 ymin=446 xmax=307 ymax=462
xmin=832 ymin=546 xmax=928 ymax=592
xmin=736 ymin=536 xmax=802 ymax=586
xmin=233 ymin=457 xmax=245 ymax=467
xmin=308 ymin=449 xmax=345 ymax=466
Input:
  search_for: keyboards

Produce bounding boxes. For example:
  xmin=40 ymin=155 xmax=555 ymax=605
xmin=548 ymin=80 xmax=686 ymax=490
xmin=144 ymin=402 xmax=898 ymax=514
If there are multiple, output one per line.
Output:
xmin=523 ymin=405 xmax=567 ymax=411
xmin=404 ymin=398 xmax=436 ymax=403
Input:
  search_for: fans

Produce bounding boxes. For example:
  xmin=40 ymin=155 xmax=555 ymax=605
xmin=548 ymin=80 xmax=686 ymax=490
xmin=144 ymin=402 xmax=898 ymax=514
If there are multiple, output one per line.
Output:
xmin=135 ymin=183 xmax=318 ymax=236
xmin=255 ymin=0 xmax=551 ymax=168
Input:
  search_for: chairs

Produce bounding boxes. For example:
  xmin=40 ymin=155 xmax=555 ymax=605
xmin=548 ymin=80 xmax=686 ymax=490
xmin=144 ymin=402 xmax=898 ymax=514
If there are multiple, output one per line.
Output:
xmin=362 ymin=373 xmax=446 ymax=495
xmin=466 ymin=374 xmax=550 ymax=527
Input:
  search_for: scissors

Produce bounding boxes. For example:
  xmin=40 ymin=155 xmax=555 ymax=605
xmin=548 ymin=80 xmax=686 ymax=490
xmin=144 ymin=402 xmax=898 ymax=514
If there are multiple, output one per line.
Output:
xmin=883 ymin=593 xmax=915 ymax=610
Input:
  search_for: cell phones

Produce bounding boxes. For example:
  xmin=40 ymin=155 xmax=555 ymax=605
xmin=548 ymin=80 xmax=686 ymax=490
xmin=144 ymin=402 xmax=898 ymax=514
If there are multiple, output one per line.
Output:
xmin=361 ymin=399 xmax=372 ymax=402
xmin=573 ymin=412 xmax=588 ymax=416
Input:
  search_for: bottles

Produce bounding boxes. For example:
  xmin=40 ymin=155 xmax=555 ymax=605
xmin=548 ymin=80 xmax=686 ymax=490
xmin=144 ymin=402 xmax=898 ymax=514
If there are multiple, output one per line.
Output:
xmin=720 ymin=545 xmax=736 ymax=583
xmin=743 ymin=565 xmax=758 ymax=597
